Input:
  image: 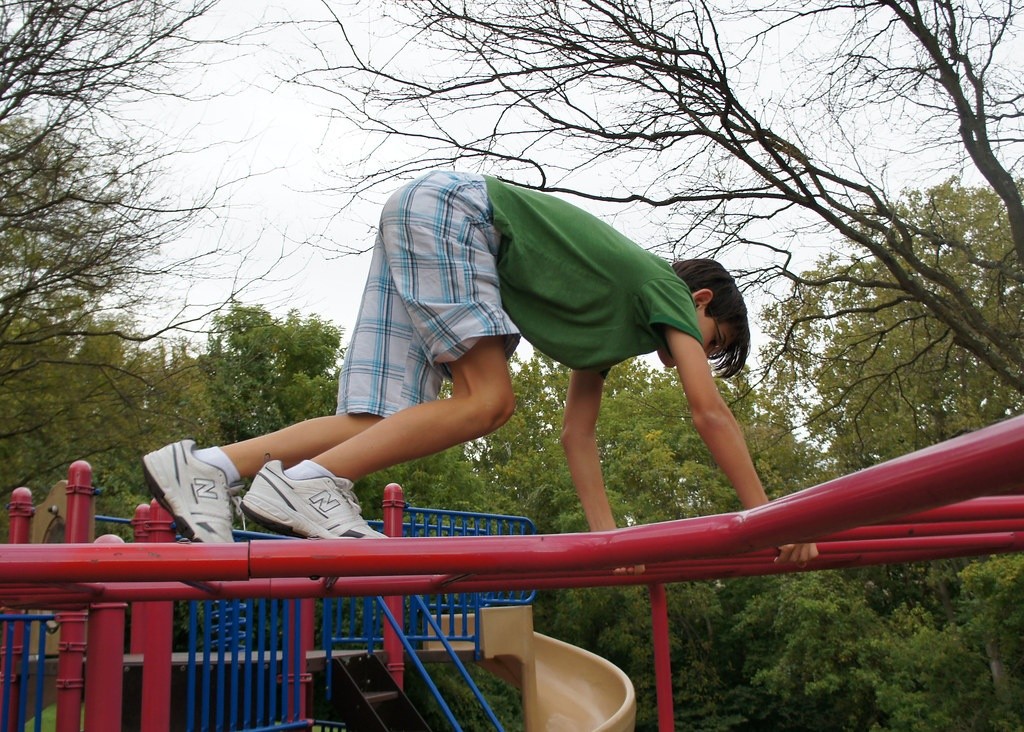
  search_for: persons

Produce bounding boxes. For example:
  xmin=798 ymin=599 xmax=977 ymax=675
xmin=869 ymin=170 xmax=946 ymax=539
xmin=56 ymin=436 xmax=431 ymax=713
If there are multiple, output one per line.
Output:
xmin=142 ymin=172 xmax=818 ymax=573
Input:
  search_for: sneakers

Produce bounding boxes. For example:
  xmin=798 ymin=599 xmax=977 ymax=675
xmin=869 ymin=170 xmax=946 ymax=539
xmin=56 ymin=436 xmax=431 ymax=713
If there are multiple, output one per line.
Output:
xmin=142 ymin=439 xmax=244 ymax=545
xmin=238 ymin=458 xmax=387 ymax=538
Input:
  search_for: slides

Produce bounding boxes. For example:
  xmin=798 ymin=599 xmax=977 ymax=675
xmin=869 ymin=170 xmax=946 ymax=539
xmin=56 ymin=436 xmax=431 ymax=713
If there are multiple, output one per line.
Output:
xmin=423 ymin=604 xmax=637 ymax=732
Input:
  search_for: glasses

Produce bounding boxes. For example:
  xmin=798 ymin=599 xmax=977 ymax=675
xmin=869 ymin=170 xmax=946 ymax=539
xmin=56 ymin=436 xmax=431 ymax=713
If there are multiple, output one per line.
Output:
xmin=707 ymin=302 xmax=726 ymax=359
